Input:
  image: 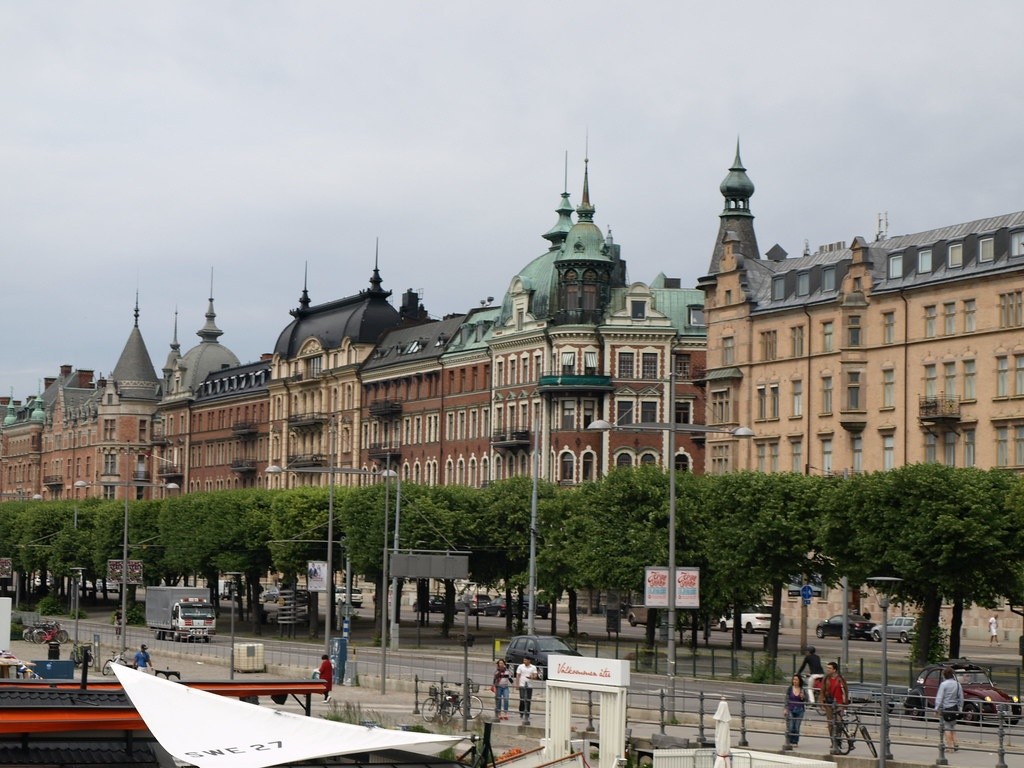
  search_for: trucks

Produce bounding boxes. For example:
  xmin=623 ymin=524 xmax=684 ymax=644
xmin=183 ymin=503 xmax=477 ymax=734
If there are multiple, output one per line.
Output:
xmin=218 ymin=580 xmax=238 ymax=601
xmin=144 ymin=585 xmax=216 ymax=643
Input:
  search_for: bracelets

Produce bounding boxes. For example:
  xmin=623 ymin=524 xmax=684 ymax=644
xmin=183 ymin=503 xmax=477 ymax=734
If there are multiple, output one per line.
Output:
xmin=150 ymin=667 xmax=153 ymax=669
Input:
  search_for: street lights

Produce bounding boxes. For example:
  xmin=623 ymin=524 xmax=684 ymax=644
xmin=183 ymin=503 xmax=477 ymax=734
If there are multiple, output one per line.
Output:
xmin=588 ymin=350 xmax=757 ymax=727
xmin=69 ymin=567 xmax=86 ymax=649
xmin=74 ymin=439 xmax=181 ymax=651
xmin=265 ymin=413 xmax=399 ymax=664
xmin=1 ymin=493 xmax=43 ymax=609
xmin=866 ymin=576 xmax=904 ymax=766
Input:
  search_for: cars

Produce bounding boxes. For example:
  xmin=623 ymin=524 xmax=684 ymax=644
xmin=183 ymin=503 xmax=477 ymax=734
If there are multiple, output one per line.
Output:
xmin=413 ymin=593 xmax=552 ymax=619
xmin=505 ymin=635 xmax=584 ymax=681
xmin=870 ymin=617 xmax=916 ymax=643
xmin=626 ymin=603 xmax=782 ymax=633
xmin=29 ymin=572 xmax=119 ymax=593
xmin=815 ymin=614 xmax=877 ymax=640
xmin=335 ymin=586 xmax=363 ymax=607
xmin=901 ymin=663 xmax=1021 ymax=726
xmin=261 ymin=586 xmax=280 ymax=604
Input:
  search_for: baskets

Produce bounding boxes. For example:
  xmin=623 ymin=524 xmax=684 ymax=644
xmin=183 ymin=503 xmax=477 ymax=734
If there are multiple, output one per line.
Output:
xmin=471 ymin=683 xmax=479 ymax=693
xmin=428 ymin=687 xmax=436 ymax=696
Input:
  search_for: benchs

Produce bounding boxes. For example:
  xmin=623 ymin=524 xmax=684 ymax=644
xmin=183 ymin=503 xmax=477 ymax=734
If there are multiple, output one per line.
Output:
xmin=586 ymin=722 xmax=632 ymax=750
xmin=634 ymin=734 xmax=689 ymax=767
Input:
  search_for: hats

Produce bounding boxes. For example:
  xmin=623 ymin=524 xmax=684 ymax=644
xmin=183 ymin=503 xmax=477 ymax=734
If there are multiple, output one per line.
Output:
xmin=141 ymin=644 xmax=148 ymax=649
xmin=806 ymin=646 xmax=815 ymax=654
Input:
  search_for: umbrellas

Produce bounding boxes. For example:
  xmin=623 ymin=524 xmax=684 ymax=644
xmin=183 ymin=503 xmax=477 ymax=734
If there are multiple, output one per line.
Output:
xmin=712 ymin=696 xmax=732 ymax=768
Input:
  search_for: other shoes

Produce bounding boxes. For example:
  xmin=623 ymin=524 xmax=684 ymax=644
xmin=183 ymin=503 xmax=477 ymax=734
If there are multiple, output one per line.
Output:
xmin=321 ymin=699 xmax=327 ymax=703
xmin=790 ymin=743 xmax=798 ymax=748
xmin=943 ymin=746 xmax=954 ymax=753
xmin=498 ymin=715 xmax=502 ymax=719
xmin=954 ymin=745 xmax=959 ymax=751
xmin=504 ymin=715 xmax=509 ymax=720
xmin=326 ymin=696 xmax=331 ymax=702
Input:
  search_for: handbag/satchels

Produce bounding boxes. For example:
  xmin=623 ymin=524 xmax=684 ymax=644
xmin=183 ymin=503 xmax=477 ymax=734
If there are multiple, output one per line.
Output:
xmin=941 ymin=705 xmax=961 ymax=722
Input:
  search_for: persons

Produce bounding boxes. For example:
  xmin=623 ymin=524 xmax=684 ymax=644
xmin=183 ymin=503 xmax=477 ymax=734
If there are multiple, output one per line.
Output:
xmin=782 ymin=673 xmax=809 ymax=750
xmin=319 ymin=655 xmax=333 ymax=704
xmin=491 ymin=659 xmax=514 ymax=721
xmin=516 ymin=656 xmax=538 ymax=720
xmin=819 ymin=662 xmax=849 ymax=748
xmin=134 ymin=643 xmax=153 ymax=674
xmin=988 ymin=614 xmax=1002 ymax=647
xmin=933 ymin=667 xmax=965 ymax=753
xmin=793 ymin=646 xmax=825 ymax=707
xmin=114 ymin=608 xmax=128 ymax=636
xmin=848 ymin=605 xmax=871 ymax=622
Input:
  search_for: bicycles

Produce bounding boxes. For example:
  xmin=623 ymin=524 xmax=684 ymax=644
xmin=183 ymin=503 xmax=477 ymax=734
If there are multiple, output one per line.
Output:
xmin=70 ymin=642 xmax=94 ymax=670
xmin=444 ymin=677 xmax=483 ymax=720
xmin=830 ymin=703 xmax=879 ymax=758
xmin=22 ymin=619 xmax=68 ymax=644
xmin=101 ymin=647 xmax=128 ymax=675
xmin=421 ymin=681 xmax=454 ymax=725
